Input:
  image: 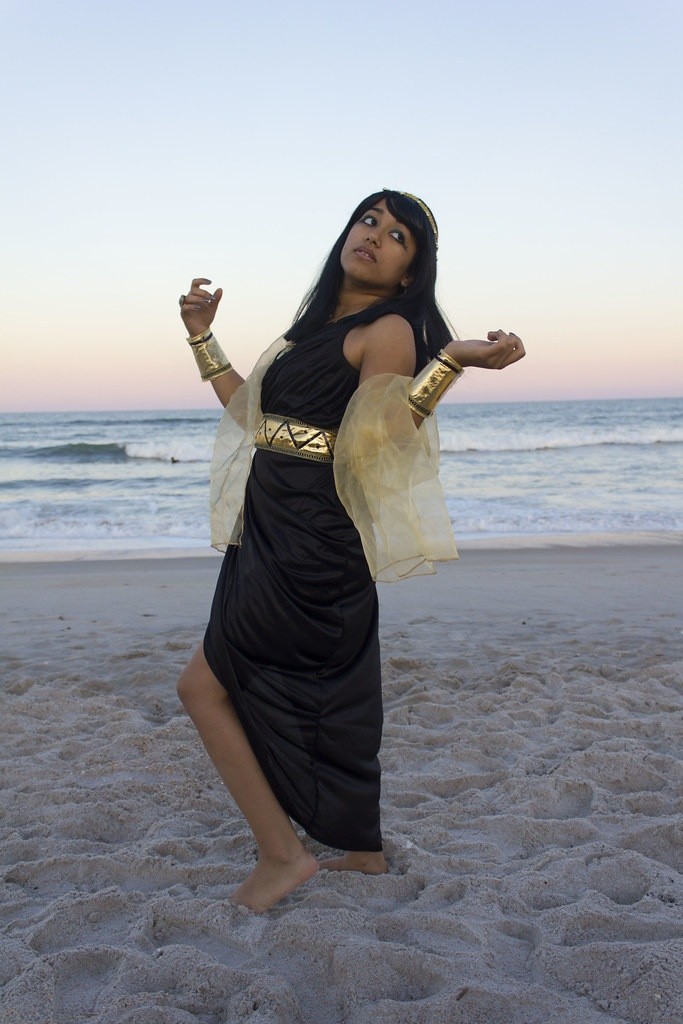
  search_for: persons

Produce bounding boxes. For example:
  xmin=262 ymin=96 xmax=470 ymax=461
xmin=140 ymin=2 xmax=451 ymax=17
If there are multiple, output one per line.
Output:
xmin=175 ymin=189 xmax=527 ymax=911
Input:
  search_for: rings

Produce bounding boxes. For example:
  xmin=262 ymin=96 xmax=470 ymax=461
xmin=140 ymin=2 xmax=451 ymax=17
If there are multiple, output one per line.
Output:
xmin=179 ymin=295 xmax=186 ymax=308
